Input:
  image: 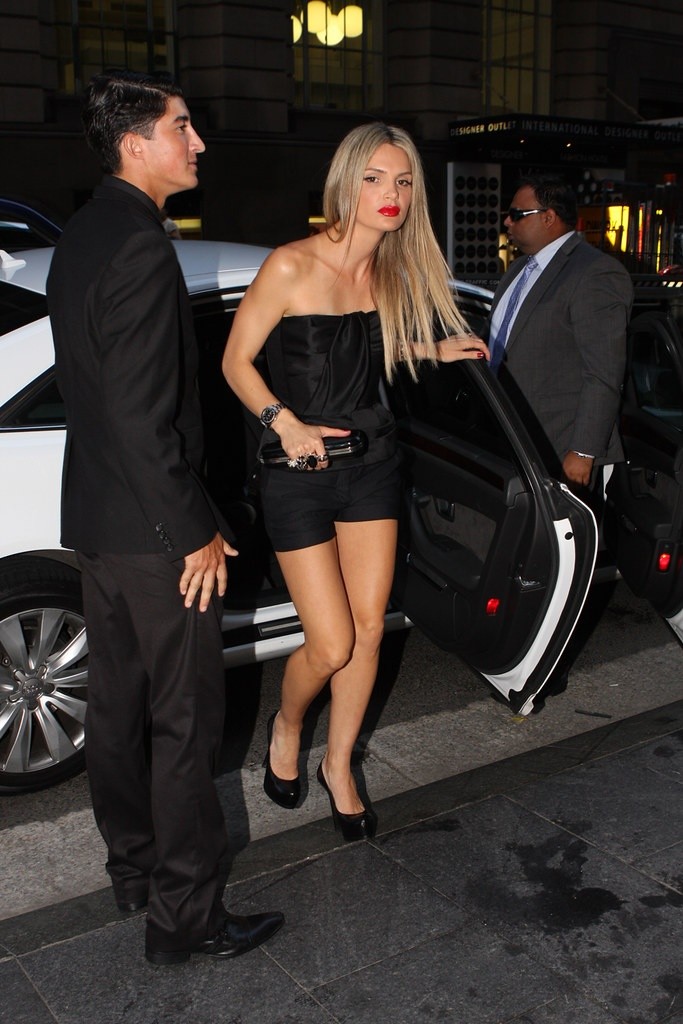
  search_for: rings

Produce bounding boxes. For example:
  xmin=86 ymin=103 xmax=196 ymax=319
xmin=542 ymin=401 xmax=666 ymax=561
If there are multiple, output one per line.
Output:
xmin=288 ymin=454 xmax=329 ymax=470
xmin=469 ymin=333 xmax=472 ymax=337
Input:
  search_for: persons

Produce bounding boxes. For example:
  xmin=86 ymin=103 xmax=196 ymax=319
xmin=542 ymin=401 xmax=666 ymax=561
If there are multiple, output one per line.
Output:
xmin=223 ymin=123 xmax=493 ymax=840
xmin=486 ymin=175 xmax=633 ymax=712
xmin=47 ymin=77 xmax=284 ymax=965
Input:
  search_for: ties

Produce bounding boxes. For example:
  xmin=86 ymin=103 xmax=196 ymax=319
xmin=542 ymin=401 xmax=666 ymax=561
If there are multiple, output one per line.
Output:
xmin=489 ymin=254 xmax=538 ymax=376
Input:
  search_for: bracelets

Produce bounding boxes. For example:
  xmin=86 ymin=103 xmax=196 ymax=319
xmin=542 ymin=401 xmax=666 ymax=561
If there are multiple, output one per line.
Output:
xmin=399 ymin=344 xmax=407 ymax=362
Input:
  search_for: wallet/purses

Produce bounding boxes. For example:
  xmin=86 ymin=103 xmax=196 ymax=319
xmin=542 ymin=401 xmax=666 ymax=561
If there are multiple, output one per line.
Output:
xmin=258 ymin=431 xmax=366 ymax=468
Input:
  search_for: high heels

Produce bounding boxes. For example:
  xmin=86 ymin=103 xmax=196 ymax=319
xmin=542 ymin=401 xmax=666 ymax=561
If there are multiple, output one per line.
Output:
xmin=262 ymin=711 xmax=302 ymax=809
xmin=316 ymin=759 xmax=374 ymax=841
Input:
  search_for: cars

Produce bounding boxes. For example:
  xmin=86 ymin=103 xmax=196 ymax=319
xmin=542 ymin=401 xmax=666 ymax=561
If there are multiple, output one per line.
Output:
xmin=0 ymin=237 xmax=679 ymax=793
xmin=0 ymin=189 xmax=67 ymax=259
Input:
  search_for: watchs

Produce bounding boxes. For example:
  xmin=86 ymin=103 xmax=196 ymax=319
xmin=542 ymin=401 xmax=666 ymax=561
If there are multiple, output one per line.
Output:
xmin=576 ymin=453 xmax=587 ymax=458
xmin=260 ymin=402 xmax=287 ymax=429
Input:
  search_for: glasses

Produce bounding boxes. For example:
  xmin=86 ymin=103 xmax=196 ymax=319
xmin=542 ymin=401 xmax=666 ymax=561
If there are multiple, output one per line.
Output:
xmin=510 ymin=207 xmax=548 ymax=221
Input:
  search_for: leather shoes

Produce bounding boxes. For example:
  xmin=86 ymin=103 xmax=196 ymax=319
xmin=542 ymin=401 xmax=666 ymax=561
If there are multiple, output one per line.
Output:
xmin=118 ymin=896 xmax=147 ymax=911
xmin=145 ymin=911 xmax=286 ymax=965
